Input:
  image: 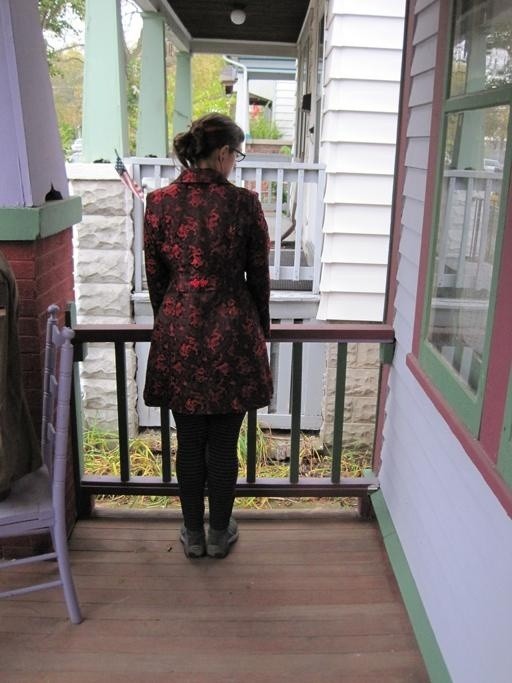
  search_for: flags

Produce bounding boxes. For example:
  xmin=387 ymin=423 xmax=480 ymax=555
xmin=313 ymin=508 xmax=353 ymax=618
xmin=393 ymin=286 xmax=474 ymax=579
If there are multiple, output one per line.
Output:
xmin=115 ymin=157 xmax=144 ymax=204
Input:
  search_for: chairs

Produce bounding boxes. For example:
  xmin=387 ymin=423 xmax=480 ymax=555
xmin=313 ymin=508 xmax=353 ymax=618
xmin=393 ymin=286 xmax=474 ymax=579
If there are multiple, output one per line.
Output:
xmin=0 ymin=304 xmax=83 ymax=632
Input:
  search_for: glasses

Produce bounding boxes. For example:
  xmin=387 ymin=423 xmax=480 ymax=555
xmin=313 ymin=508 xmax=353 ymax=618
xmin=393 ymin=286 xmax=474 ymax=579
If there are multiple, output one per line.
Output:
xmin=219 ymin=144 xmax=246 ymax=162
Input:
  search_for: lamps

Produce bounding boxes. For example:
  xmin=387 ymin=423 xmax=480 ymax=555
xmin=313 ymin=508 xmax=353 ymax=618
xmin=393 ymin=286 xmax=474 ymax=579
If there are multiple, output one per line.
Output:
xmin=301 ymin=94 xmax=311 ymax=112
xmin=230 ymin=3 xmax=247 ymax=25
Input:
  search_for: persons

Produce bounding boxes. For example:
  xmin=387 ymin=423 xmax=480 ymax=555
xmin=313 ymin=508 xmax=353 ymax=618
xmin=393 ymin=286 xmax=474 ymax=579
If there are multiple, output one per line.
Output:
xmin=140 ymin=112 xmax=274 ymax=557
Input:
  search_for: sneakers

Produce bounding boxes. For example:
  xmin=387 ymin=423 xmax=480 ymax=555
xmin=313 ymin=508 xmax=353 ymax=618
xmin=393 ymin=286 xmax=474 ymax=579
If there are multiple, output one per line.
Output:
xmin=207 ymin=516 xmax=240 ymax=558
xmin=178 ymin=518 xmax=207 ymax=556
